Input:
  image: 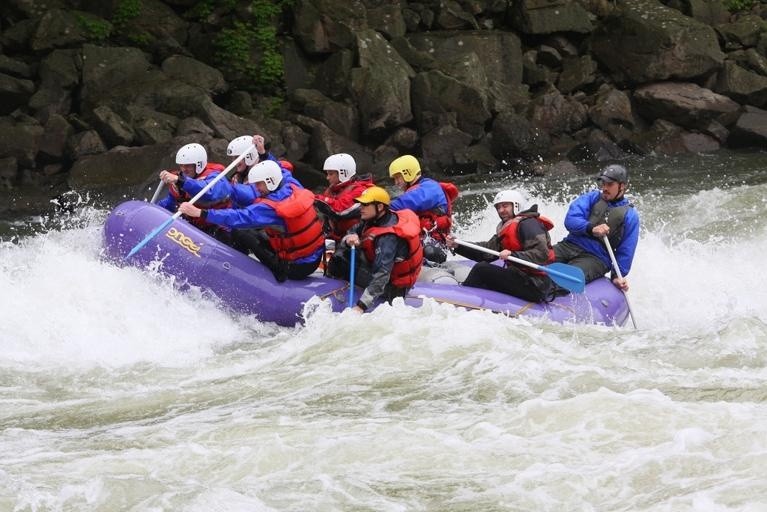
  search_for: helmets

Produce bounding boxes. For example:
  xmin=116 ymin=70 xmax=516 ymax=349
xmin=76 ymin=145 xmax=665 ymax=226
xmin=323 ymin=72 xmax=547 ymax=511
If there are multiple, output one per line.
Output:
xmin=248 ymin=160 xmax=284 ymax=191
xmin=175 ymin=143 xmax=208 ymax=175
xmin=323 ymin=153 xmax=357 ymax=184
xmin=353 ymin=186 xmax=391 ymax=207
xmin=597 ymin=164 xmax=627 ymax=184
xmin=493 ymin=190 xmax=524 ymax=219
xmin=226 ymin=135 xmax=260 ymax=167
xmin=389 ymin=154 xmax=422 ymax=183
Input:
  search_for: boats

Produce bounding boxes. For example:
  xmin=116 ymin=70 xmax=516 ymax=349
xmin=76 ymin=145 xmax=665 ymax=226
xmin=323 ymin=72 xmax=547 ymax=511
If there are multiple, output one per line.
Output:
xmin=104 ymin=199 xmax=630 ymax=329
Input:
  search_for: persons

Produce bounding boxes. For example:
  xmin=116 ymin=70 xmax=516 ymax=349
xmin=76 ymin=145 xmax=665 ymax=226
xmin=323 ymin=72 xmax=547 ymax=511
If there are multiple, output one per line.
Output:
xmin=552 ymin=165 xmax=640 ymax=297
xmin=156 ymin=142 xmax=232 ymax=247
xmin=388 ymin=154 xmax=458 ymax=267
xmin=178 ymin=134 xmax=325 ymax=281
xmin=315 ymin=152 xmax=377 ymax=271
xmin=446 ymin=190 xmax=556 ymax=304
xmin=325 ymin=186 xmax=424 ymax=314
xmin=227 ymin=135 xmax=294 ymax=256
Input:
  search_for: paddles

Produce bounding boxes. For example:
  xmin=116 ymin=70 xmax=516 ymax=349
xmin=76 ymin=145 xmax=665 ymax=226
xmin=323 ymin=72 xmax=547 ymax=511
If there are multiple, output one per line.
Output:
xmin=451 ymin=238 xmax=585 ymax=294
xmin=127 ymin=143 xmax=256 ymax=257
